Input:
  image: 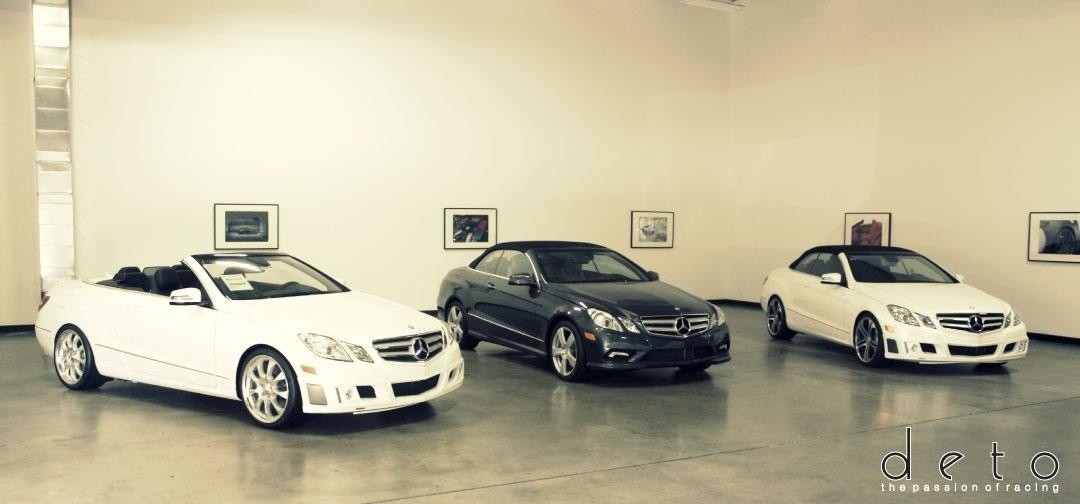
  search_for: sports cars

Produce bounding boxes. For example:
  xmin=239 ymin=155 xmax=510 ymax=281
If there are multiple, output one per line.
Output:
xmin=757 ymin=243 xmax=1030 ymax=376
xmin=32 ymin=248 xmax=466 ymax=429
xmin=435 ymin=239 xmax=733 ymax=386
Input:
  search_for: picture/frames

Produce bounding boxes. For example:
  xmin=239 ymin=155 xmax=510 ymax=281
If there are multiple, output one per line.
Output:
xmin=443 ymin=207 xmax=497 ymax=248
xmin=1027 ymin=210 xmax=1080 ymax=263
xmin=630 ymin=210 xmax=674 ymax=248
xmin=214 ymin=202 xmax=279 ymax=249
xmin=844 ymin=212 xmax=891 ymax=247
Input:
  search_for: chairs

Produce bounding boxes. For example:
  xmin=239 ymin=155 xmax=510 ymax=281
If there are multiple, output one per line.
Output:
xmin=149 ymin=264 xmax=247 ymax=296
